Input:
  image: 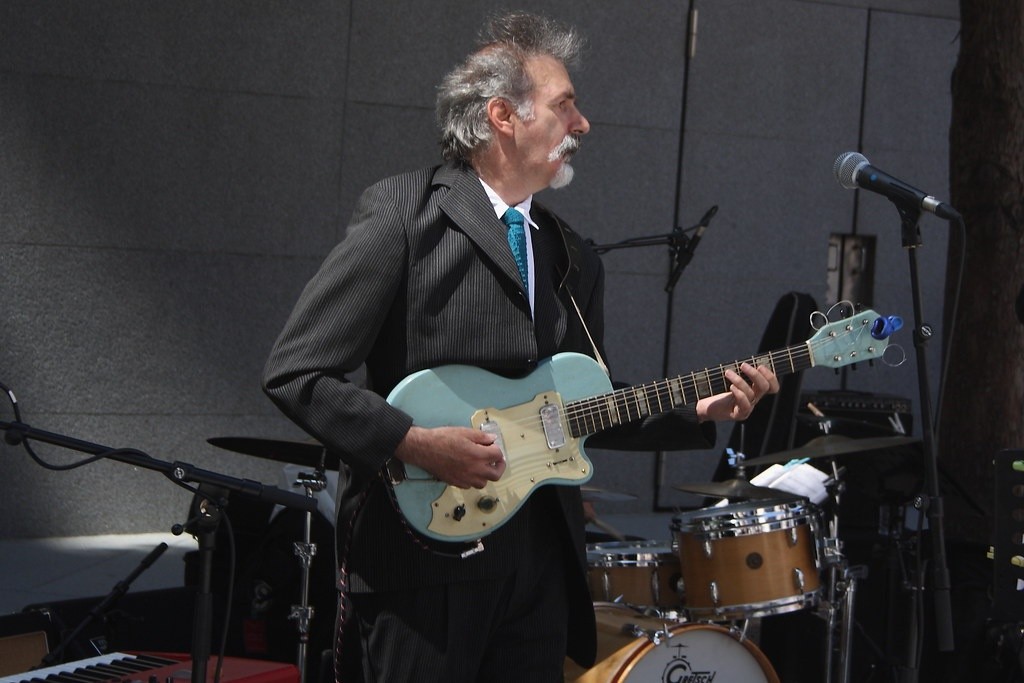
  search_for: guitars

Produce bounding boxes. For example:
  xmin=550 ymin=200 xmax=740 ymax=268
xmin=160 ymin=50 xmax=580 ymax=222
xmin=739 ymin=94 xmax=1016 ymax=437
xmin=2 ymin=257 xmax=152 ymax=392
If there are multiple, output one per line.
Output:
xmin=379 ymin=300 xmax=908 ymax=560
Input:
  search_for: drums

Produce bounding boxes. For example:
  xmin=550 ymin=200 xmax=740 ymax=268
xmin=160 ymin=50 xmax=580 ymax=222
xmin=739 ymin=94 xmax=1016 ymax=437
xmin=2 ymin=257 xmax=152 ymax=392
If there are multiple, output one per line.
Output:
xmin=668 ymin=494 xmax=825 ymax=622
xmin=585 ymin=539 xmax=681 ymax=612
xmin=561 ymin=600 xmax=780 ymax=683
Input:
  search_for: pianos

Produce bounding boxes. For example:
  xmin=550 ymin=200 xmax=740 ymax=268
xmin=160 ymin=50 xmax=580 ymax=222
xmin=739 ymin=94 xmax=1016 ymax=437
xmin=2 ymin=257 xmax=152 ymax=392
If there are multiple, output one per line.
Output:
xmin=0 ymin=649 xmax=301 ymax=683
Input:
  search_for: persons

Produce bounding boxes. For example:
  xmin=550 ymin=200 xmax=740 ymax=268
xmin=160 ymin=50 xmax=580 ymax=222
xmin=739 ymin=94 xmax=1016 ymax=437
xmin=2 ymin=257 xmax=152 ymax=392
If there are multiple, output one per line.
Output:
xmin=259 ymin=7 xmax=781 ymax=682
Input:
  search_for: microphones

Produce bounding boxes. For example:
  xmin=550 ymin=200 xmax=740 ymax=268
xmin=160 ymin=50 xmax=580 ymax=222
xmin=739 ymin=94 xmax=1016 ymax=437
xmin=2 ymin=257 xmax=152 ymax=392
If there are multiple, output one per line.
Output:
xmin=832 ymin=152 xmax=961 ymax=221
xmin=665 ymin=209 xmax=713 ymax=294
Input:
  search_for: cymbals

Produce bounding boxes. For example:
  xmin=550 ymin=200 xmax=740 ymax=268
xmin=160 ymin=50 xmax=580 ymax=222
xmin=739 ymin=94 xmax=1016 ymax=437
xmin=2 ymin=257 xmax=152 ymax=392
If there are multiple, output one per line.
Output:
xmin=670 ymin=479 xmax=797 ymax=498
xmin=732 ymin=433 xmax=923 ymax=466
xmin=206 ymin=436 xmax=342 ymax=471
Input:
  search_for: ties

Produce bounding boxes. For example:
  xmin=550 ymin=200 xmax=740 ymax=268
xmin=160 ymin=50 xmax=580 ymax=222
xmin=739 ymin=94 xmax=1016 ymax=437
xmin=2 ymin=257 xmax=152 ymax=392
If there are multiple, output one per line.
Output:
xmin=502 ymin=207 xmax=528 ymax=294
